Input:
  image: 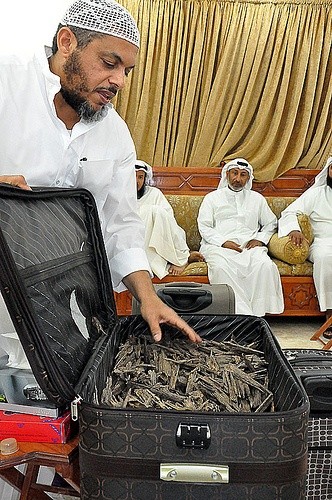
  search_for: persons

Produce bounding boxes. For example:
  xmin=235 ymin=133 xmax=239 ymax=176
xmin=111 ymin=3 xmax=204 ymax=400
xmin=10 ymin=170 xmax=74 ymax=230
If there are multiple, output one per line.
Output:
xmin=0 ymin=0 xmax=202 ymax=368
xmin=196 ymin=158 xmax=285 ymax=318
xmin=135 ymin=159 xmax=203 ymax=279
xmin=278 ymin=157 xmax=332 ymax=339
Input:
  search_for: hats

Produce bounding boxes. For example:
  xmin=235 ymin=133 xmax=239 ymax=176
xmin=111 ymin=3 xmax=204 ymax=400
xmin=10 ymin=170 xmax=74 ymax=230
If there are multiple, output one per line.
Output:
xmin=60 ymin=0 xmax=140 ymax=48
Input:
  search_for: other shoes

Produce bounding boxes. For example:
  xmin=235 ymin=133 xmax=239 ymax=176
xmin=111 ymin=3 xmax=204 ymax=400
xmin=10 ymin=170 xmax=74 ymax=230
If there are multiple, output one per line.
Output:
xmin=323 ymin=327 xmax=332 ymax=339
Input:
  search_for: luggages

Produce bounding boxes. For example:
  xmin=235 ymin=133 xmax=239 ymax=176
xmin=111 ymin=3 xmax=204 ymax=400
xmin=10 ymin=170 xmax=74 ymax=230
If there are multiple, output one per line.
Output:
xmin=132 ymin=282 xmax=236 ymax=315
xmin=0 ymin=181 xmax=310 ymax=500
xmin=282 ymin=349 xmax=332 ymax=500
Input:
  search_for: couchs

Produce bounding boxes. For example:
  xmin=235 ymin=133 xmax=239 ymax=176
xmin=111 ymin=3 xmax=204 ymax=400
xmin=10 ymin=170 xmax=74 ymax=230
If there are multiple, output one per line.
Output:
xmin=113 ymin=166 xmax=332 ymax=316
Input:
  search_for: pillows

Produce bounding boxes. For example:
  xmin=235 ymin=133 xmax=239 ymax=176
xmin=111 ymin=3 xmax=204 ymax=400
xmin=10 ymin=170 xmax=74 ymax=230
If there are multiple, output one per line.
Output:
xmin=297 ymin=214 xmax=314 ymax=245
xmin=267 ymin=233 xmax=310 ymax=264
